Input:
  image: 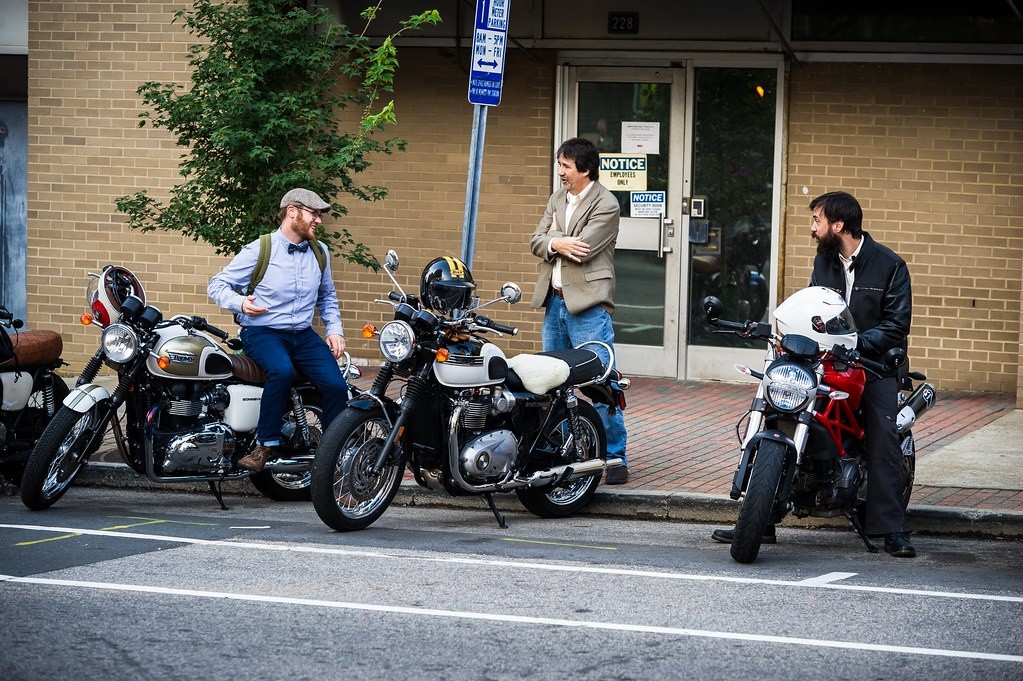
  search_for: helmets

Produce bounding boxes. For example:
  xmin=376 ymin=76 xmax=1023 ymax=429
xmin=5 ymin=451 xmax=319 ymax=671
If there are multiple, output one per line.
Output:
xmin=773 ymin=286 xmax=858 ymax=351
xmin=419 ymin=255 xmax=476 ymax=310
xmin=86 ymin=264 xmax=147 ymax=329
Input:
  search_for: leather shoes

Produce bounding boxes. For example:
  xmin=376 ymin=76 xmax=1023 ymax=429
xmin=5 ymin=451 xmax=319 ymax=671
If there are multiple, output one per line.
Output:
xmin=885 ymin=529 xmax=915 ymax=556
xmin=712 ymin=519 xmax=776 ymax=543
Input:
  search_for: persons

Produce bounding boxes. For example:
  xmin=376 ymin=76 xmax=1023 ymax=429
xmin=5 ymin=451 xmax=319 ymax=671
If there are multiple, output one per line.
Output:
xmin=711 ymin=191 xmax=917 ymax=558
xmin=206 ymin=187 xmax=347 ymax=507
xmin=529 ymin=136 xmax=629 ymax=485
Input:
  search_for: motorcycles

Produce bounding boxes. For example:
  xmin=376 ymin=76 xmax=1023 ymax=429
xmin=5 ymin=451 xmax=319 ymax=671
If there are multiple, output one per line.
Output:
xmin=591 ymin=175 xmax=769 ymax=346
xmin=20 ymin=265 xmax=342 ymax=511
xmin=699 ymin=295 xmax=936 ymax=564
xmin=0 ymin=306 xmax=72 ymax=499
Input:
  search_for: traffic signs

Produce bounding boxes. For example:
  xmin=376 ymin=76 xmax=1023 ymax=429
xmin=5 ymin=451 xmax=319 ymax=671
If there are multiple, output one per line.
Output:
xmin=467 ymin=0 xmax=511 ymax=107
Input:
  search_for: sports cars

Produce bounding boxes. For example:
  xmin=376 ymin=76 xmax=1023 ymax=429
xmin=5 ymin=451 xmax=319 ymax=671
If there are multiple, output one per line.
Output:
xmin=310 ymin=252 xmax=631 ymax=534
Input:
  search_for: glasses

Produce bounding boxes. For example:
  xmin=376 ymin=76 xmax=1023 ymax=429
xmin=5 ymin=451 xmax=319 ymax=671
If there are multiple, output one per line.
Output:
xmin=293 ymin=205 xmax=323 ymax=221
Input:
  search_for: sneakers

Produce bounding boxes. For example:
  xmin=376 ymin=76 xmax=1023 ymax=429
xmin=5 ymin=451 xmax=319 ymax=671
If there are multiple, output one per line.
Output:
xmin=341 ymin=492 xmax=356 ymax=508
xmin=239 ymin=445 xmax=267 ymax=471
xmin=606 ymin=464 xmax=628 ymax=484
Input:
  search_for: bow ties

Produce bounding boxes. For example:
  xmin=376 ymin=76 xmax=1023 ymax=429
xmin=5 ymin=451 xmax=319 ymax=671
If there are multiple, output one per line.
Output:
xmin=288 ymin=244 xmax=308 ymax=255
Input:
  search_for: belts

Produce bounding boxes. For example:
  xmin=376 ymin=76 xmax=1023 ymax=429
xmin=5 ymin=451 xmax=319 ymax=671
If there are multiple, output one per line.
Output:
xmin=547 ymin=288 xmax=564 ymax=299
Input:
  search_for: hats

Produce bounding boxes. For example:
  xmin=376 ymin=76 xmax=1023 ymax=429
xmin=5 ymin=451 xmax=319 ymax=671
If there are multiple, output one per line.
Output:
xmin=280 ymin=188 xmax=331 ymax=213
